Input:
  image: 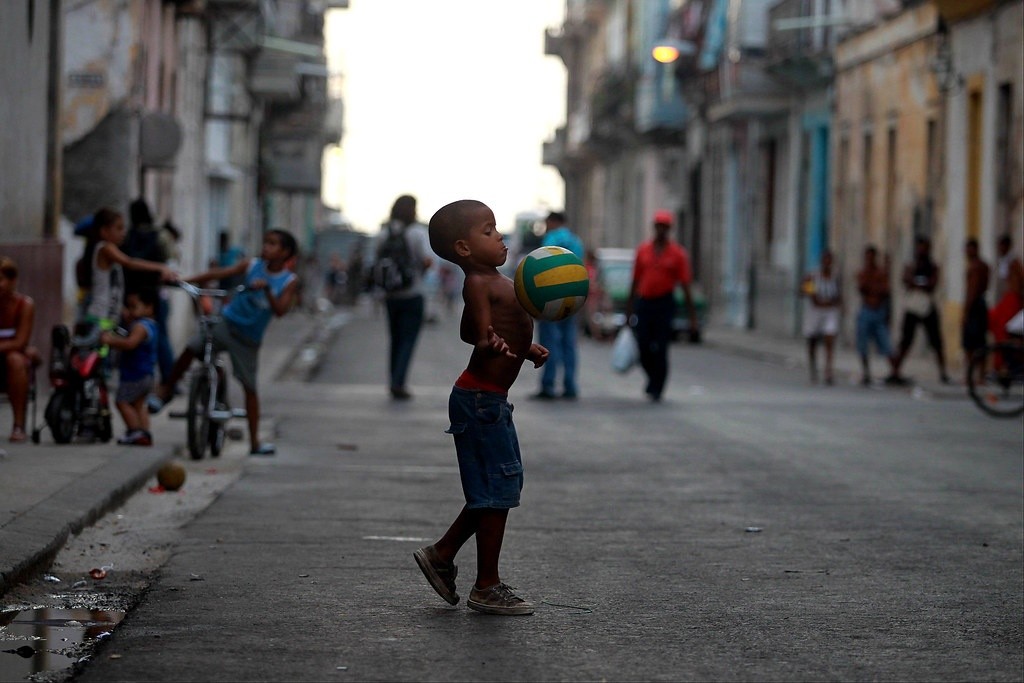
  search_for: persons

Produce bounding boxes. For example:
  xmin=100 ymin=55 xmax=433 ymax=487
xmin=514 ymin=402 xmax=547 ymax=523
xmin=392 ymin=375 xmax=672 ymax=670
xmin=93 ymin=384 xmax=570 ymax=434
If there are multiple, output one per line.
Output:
xmin=0 ymin=257 xmax=43 ymax=443
xmin=76 ymin=201 xmax=300 ymax=455
xmin=625 ymin=212 xmax=697 ymax=397
xmin=800 ymin=236 xmax=949 ymax=387
xmin=414 ymin=199 xmax=548 ymax=614
xmin=539 ymin=212 xmax=599 ymax=400
xmin=305 ymin=196 xmax=457 ymax=397
xmin=962 ymin=233 xmax=1024 ymax=384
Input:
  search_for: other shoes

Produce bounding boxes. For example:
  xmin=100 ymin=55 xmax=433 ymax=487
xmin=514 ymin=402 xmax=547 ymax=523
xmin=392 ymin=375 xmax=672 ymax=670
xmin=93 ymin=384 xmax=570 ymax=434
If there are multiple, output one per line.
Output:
xmin=862 ymin=375 xmax=870 ymax=385
xmin=942 ymin=374 xmax=950 ymax=384
xmin=390 ymin=385 xmax=410 ymax=399
xmin=562 ymin=390 xmax=577 ymax=398
xmin=9 ymin=424 xmax=25 ymax=442
xmin=250 ymin=444 xmax=275 ymax=455
xmin=530 ymin=391 xmax=554 ymax=399
xmin=885 ymin=375 xmax=907 ymax=386
xmin=118 ymin=428 xmax=151 ymax=445
xmin=145 ymin=393 xmax=162 ymax=412
xmin=647 ymin=382 xmax=662 ymax=399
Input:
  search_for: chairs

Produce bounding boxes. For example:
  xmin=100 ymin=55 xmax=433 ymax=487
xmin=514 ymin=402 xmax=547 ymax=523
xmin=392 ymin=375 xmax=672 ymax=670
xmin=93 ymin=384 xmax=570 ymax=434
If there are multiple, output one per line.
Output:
xmin=0 ymin=355 xmax=44 ymax=439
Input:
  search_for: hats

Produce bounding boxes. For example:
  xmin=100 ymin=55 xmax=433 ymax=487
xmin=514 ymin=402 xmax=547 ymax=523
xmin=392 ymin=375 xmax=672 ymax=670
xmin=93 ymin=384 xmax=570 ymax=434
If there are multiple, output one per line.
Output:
xmin=653 ymin=209 xmax=673 ymax=225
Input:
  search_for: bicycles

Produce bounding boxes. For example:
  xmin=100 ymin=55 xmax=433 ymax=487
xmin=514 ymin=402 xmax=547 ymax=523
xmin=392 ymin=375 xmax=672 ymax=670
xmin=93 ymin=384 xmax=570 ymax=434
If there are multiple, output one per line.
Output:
xmin=966 ymin=329 xmax=1023 ymax=418
xmin=30 ymin=315 xmax=129 ymax=446
xmin=168 ymin=273 xmax=249 ymax=462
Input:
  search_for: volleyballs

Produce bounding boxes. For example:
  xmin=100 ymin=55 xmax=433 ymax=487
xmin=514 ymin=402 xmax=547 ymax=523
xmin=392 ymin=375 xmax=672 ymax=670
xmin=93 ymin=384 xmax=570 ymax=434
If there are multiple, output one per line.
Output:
xmin=514 ymin=246 xmax=590 ymax=324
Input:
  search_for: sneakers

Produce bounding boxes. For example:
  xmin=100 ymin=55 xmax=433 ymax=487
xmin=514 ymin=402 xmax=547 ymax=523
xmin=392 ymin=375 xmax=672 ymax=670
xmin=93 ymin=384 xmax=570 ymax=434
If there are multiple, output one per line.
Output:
xmin=414 ymin=545 xmax=460 ymax=606
xmin=467 ymin=583 xmax=535 ymax=614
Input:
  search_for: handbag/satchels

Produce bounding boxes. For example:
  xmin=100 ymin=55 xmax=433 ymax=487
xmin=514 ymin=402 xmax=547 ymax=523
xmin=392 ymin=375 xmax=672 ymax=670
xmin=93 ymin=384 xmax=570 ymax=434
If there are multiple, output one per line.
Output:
xmin=903 ymin=288 xmax=932 ymax=315
xmin=614 ymin=324 xmax=639 ymax=372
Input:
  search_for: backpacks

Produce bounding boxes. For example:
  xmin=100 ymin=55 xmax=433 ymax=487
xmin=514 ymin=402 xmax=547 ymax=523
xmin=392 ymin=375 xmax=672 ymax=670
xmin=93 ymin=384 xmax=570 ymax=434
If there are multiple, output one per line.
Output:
xmin=370 ymin=223 xmax=414 ymax=290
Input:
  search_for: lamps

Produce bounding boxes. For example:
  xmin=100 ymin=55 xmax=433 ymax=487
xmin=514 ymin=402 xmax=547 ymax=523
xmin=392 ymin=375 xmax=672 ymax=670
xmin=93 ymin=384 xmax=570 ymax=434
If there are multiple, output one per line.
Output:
xmin=928 ymin=43 xmax=966 ymax=97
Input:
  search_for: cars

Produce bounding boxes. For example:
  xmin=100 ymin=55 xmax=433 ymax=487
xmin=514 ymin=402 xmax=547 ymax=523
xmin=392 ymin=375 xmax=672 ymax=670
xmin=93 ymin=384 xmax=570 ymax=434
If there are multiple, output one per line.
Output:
xmin=584 ymin=247 xmax=707 ymax=340
